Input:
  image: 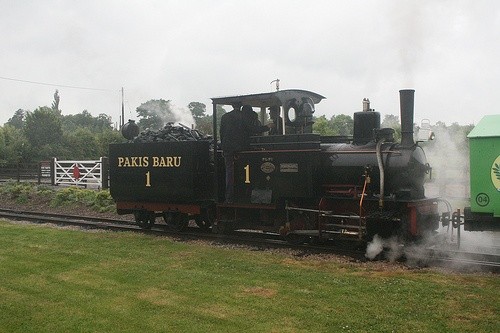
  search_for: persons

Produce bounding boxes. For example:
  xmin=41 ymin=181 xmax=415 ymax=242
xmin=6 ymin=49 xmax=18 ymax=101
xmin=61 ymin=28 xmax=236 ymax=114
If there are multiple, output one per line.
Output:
xmin=265 ymin=105 xmax=283 ymax=135
xmin=219 ymin=102 xmax=274 ymax=204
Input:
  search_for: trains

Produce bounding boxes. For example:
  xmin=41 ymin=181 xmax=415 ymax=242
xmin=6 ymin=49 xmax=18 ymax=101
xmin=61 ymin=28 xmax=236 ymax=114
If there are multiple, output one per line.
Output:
xmin=108 ymin=77 xmax=467 ymax=252
